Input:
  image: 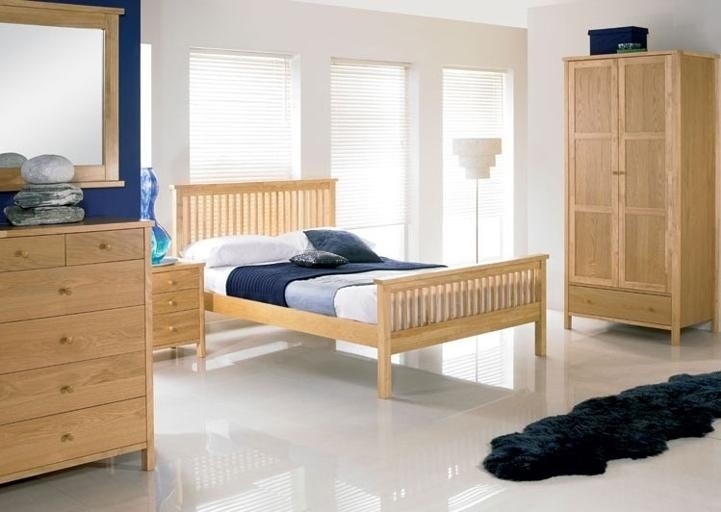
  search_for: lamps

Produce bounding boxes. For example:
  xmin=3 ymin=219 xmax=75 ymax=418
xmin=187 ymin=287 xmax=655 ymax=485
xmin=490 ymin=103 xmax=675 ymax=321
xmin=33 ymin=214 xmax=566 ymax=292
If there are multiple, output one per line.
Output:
xmin=451 ymin=138 xmax=503 ymax=263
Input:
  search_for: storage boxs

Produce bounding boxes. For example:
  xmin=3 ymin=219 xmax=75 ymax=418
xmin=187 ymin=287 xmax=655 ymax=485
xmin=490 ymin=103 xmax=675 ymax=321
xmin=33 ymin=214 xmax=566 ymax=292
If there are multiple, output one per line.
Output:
xmin=587 ymin=25 xmax=649 ymax=55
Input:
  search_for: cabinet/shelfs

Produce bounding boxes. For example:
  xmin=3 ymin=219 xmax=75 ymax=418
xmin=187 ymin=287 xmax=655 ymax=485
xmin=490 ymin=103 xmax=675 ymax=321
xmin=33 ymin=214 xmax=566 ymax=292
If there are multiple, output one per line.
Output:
xmin=0 ymin=215 xmax=157 ymax=486
xmin=561 ymin=48 xmax=721 ymax=347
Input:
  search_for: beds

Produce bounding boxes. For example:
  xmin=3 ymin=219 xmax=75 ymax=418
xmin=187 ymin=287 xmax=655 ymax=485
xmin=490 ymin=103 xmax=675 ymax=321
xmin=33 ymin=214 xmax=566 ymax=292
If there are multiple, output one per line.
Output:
xmin=171 ymin=178 xmax=550 ymax=400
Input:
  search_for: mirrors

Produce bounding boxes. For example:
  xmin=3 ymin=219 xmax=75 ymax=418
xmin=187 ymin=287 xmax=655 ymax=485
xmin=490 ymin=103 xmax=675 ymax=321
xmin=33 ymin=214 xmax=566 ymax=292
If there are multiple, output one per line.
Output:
xmin=0 ymin=0 xmax=125 ymax=194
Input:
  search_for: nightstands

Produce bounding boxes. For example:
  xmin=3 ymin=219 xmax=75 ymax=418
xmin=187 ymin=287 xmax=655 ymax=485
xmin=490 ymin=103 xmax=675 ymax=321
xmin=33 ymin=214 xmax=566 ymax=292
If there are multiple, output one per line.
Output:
xmin=151 ymin=257 xmax=207 ymax=359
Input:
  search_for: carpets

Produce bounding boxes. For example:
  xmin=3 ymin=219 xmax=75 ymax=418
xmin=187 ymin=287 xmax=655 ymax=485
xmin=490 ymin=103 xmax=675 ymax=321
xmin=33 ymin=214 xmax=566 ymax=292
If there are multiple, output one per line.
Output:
xmin=480 ymin=370 xmax=721 ymax=482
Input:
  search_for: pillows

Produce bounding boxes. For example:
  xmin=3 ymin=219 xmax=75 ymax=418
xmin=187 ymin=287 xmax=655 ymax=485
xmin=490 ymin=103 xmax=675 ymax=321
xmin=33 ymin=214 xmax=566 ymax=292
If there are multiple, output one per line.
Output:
xmin=179 ymin=226 xmax=385 ymax=268
